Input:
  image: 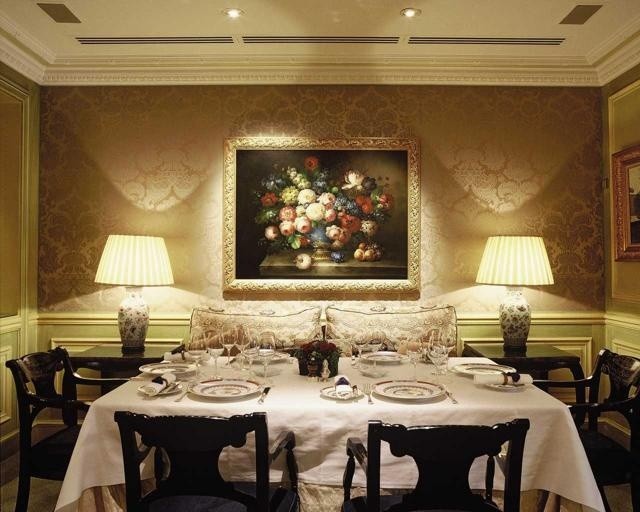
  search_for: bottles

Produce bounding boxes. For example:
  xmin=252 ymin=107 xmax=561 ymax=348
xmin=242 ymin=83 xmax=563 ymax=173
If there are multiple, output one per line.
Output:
xmin=320 ymin=359 xmax=331 ymax=383
xmin=307 ymin=357 xmax=318 ymax=377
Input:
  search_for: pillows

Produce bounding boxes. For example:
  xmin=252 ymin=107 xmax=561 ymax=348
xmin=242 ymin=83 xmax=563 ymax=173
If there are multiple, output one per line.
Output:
xmin=185 ymin=301 xmax=322 ymax=360
xmin=324 ymin=304 xmax=458 ymax=361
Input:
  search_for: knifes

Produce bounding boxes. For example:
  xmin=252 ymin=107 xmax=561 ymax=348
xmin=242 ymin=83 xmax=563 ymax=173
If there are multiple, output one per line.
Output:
xmin=162 ymin=379 xmax=184 ymax=395
xmin=257 ymin=386 xmax=271 ymax=404
xmin=440 ymin=385 xmax=460 ymax=407
xmin=353 ymin=384 xmax=358 ymax=399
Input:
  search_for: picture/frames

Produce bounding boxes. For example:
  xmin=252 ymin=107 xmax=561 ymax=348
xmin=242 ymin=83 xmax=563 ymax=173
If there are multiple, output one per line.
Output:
xmin=223 ymin=137 xmax=420 ymax=293
xmin=612 ymin=144 xmax=640 ymax=262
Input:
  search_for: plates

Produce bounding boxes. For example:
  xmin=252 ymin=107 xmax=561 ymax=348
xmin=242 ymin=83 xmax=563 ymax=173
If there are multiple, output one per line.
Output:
xmin=488 ymin=385 xmax=527 ymax=392
xmin=138 ymin=362 xmax=203 ymax=376
xmin=452 ymin=362 xmax=519 ymax=377
xmin=363 ymin=350 xmax=409 ymax=364
xmin=236 ymin=349 xmax=292 ymax=363
xmin=320 ymin=386 xmax=362 ymax=402
xmin=188 ymin=378 xmax=261 ymax=399
xmin=370 ymin=377 xmax=446 ymax=401
xmin=137 ymin=383 xmax=182 ymax=397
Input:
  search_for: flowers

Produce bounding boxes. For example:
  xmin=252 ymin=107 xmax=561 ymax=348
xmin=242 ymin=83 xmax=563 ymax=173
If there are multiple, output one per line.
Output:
xmin=291 ymin=340 xmax=344 ymax=378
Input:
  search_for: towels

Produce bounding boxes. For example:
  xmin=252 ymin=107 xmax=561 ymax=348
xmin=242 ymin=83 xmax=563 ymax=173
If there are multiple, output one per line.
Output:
xmin=144 ymin=373 xmax=176 ymax=397
xmin=163 ymin=345 xmax=191 ymax=361
xmin=474 ymin=373 xmax=533 ymax=385
xmin=335 ymin=375 xmax=353 ymax=400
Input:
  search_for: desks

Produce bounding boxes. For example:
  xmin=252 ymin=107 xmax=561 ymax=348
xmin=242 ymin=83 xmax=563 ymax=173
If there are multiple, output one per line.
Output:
xmin=461 ymin=343 xmax=585 ymax=429
xmin=62 ymin=346 xmax=181 ymax=426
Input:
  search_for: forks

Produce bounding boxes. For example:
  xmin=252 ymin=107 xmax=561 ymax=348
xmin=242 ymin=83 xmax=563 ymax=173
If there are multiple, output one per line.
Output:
xmin=363 ymin=382 xmax=374 ymax=407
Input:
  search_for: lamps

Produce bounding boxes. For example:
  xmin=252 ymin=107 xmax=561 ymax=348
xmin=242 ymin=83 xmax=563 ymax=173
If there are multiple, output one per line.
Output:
xmin=475 ymin=236 xmax=555 ymax=353
xmin=94 ymin=234 xmax=174 ymax=353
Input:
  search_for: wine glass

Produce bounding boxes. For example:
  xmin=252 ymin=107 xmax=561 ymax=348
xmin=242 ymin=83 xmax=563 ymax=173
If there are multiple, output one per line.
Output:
xmin=351 ymin=325 xmax=382 ymax=374
xmin=188 ymin=323 xmax=276 ymax=386
xmin=405 ymin=324 xmax=458 ymax=383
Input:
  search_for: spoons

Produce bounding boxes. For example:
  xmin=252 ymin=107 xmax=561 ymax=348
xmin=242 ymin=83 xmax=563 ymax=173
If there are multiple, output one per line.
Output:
xmin=174 ymin=387 xmax=192 ymax=403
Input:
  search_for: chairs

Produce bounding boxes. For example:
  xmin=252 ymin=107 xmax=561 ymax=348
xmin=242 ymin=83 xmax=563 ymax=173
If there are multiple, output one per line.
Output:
xmin=5 ymin=346 xmax=130 ymax=512
xmin=341 ymin=418 xmax=530 ymax=511
xmin=533 ymin=347 xmax=640 ymax=511
xmin=114 ymin=411 xmax=300 ymax=512
